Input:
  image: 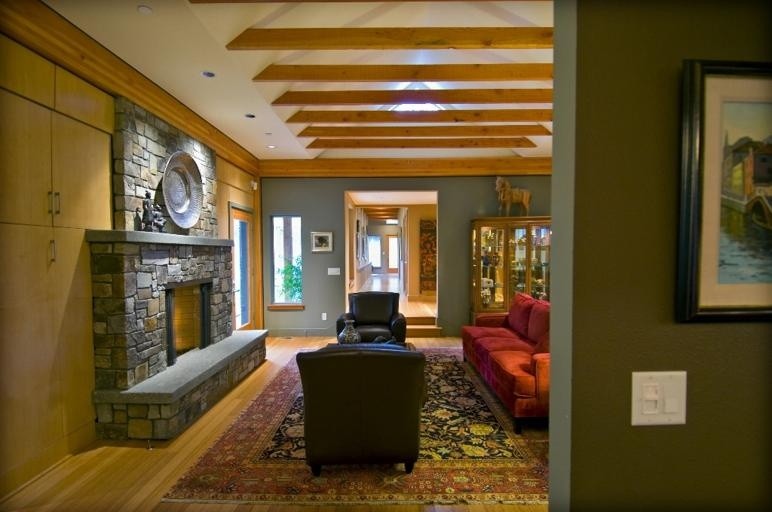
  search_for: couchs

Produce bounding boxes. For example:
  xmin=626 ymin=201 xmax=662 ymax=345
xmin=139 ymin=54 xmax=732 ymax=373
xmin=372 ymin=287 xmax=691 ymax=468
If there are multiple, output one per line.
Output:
xmin=461 ymin=313 xmax=551 ymax=434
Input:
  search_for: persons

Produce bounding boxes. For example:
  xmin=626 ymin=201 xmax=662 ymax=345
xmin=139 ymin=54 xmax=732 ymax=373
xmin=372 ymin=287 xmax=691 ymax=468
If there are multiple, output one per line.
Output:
xmin=142 ymin=191 xmax=166 ymax=232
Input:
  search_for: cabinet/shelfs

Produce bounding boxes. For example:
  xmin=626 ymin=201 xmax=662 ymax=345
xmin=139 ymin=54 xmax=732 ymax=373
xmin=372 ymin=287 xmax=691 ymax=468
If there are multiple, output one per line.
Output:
xmin=0 ymin=34 xmax=114 ymax=503
xmin=469 ymin=216 xmax=552 ymax=325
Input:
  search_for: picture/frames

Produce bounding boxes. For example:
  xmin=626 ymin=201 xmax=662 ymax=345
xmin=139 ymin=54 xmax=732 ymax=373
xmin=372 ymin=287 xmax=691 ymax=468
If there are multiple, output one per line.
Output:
xmin=672 ymin=59 xmax=772 ymax=324
xmin=311 ymin=232 xmax=332 ymax=251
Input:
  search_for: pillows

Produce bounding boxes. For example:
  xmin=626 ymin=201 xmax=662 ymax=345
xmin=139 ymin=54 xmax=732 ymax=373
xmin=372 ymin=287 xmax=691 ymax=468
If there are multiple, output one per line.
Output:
xmin=509 ymin=291 xmax=550 ymax=372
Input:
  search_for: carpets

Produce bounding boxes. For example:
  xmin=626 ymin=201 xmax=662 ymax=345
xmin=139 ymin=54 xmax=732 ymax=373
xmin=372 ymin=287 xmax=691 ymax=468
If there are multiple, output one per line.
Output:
xmin=160 ymin=345 xmax=548 ymax=506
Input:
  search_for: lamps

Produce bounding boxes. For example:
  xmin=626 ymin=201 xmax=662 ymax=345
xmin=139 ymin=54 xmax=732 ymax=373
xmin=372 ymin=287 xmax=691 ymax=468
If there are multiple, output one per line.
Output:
xmin=377 ymin=82 xmax=457 ymax=111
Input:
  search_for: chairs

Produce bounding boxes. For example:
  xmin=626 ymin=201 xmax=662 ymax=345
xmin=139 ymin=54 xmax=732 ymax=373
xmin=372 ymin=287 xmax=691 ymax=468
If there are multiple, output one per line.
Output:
xmin=336 ymin=291 xmax=406 ymax=343
xmin=296 ymin=351 xmax=425 ymax=473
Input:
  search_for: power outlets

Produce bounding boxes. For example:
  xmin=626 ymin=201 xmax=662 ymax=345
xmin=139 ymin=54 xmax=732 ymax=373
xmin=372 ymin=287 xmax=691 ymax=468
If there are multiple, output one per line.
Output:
xmin=631 ymin=371 xmax=687 ymax=426
